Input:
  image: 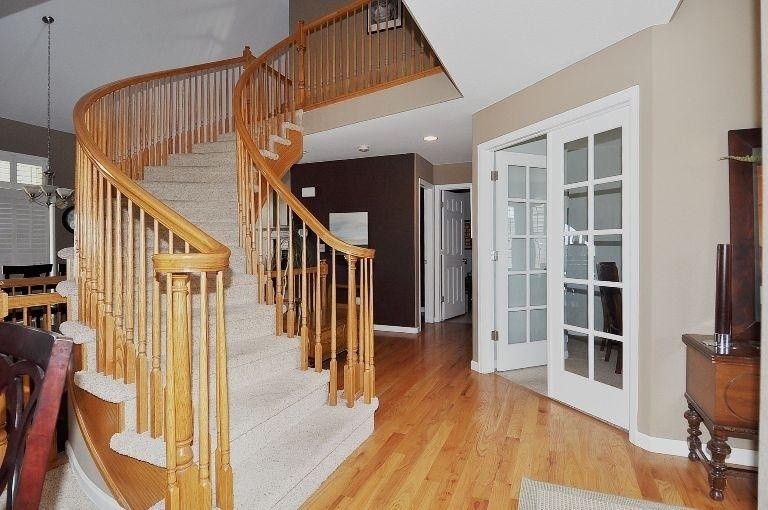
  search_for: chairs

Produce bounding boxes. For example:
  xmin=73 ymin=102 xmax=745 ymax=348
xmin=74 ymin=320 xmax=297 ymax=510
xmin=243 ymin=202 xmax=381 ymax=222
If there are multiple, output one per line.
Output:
xmin=0 ymin=264 xmax=73 ymax=510
xmin=596 ymin=262 xmax=622 ymax=374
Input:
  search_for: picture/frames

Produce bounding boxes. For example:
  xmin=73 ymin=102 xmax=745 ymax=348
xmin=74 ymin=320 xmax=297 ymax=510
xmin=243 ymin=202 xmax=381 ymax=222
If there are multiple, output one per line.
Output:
xmin=367 ymin=0 xmax=403 ymax=35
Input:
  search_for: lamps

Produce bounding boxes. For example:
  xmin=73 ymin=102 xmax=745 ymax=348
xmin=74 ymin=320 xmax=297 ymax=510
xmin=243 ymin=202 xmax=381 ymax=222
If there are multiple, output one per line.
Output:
xmin=23 ymin=15 xmax=75 ymax=209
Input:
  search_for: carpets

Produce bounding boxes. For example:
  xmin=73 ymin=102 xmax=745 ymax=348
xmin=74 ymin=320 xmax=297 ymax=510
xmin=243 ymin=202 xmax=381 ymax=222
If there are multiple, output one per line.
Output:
xmin=517 ymin=477 xmax=690 ymax=510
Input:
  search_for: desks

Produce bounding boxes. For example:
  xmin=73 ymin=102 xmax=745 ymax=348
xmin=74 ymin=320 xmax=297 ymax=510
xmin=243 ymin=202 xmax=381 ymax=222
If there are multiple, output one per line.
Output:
xmin=682 ymin=334 xmax=760 ymax=502
